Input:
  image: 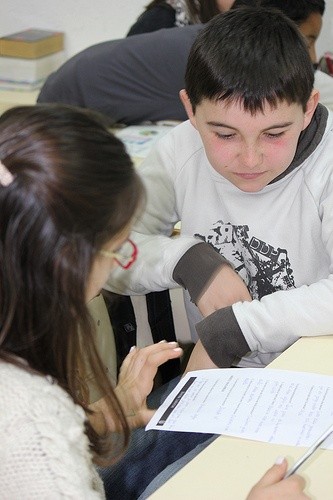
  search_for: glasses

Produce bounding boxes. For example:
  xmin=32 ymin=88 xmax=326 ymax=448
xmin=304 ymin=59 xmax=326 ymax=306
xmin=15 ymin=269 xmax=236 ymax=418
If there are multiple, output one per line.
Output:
xmin=98 ymin=239 xmax=137 ymax=269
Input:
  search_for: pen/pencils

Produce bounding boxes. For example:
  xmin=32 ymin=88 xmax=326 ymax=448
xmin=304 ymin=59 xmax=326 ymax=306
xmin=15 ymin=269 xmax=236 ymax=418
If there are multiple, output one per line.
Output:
xmin=280 ymin=426 xmax=332 ymax=480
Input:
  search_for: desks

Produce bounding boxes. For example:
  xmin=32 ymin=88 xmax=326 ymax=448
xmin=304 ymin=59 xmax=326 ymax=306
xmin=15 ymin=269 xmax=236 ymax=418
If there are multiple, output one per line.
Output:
xmin=111 ymin=124 xmax=181 ymax=163
xmin=145 ymin=336 xmax=333 ymax=499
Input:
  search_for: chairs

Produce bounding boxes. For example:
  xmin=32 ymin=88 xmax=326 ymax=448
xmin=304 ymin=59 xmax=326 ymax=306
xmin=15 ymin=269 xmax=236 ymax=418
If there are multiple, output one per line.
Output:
xmin=82 ymin=288 xmax=194 ymax=403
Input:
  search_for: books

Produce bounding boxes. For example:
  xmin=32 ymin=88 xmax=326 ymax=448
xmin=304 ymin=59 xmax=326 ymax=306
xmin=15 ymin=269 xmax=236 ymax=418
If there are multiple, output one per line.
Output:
xmin=0 ymin=29 xmax=64 ymax=60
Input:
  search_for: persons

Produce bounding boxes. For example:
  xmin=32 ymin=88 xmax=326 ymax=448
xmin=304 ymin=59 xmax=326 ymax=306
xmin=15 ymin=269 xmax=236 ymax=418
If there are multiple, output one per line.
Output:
xmin=36 ymin=0 xmax=326 ymax=124
xmin=102 ymin=7 xmax=333 ymax=377
xmin=0 ymin=105 xmax=310 ymax=500
